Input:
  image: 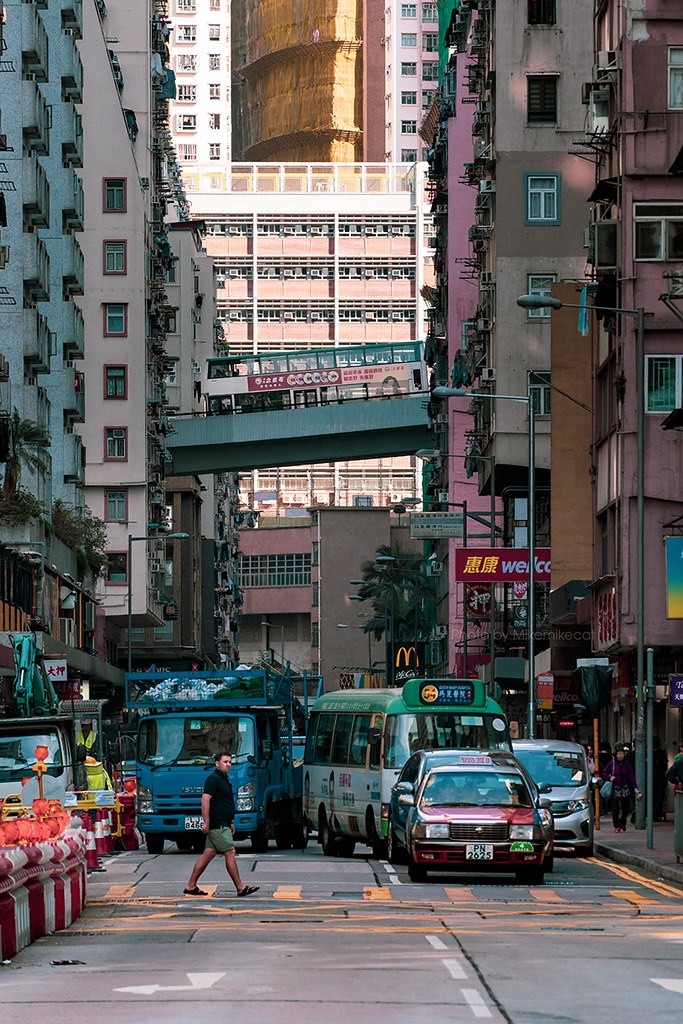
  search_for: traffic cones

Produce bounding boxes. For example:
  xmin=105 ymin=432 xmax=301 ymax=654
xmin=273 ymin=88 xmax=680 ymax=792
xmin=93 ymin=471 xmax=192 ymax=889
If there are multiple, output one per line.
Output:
xmin=94 ymin=810 xmax=112 ymax=858
xmin=85 ymin=819 xmax=109 ymax=873
xmin=102 ymin=808 xmax=122 ymax=856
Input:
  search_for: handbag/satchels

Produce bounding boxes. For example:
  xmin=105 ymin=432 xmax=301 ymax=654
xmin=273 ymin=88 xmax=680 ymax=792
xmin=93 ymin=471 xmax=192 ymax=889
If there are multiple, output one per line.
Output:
xmin=600 ymin=780 xmax=612 ymax=799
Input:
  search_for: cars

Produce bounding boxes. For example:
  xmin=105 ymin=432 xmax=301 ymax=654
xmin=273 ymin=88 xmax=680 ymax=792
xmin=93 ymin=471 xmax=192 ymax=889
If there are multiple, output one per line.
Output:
xmin=399 ymin=757 xmax=548 ymax=885
xmin=510 ymin=740 xmax=608 ymax=850
xmin=388 ymin=748 xmax=558 ymax=874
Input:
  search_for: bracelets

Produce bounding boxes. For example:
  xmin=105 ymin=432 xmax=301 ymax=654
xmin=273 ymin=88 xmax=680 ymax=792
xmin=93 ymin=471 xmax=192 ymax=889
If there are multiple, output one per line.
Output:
xmin=230 ymin=822 xmax=233 ymax=824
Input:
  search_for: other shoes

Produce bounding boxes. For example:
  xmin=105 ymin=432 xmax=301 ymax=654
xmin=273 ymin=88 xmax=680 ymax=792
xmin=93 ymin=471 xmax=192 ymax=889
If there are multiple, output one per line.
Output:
xmin=614 ymin=825 xmax=627 ymax=834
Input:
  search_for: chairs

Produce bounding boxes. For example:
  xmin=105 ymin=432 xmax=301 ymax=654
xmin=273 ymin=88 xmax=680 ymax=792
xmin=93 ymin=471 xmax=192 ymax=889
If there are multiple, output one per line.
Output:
xmin=434 ymin=781 xmax=461 ymax=800
xmin=487 ymin=790 xmax=510 ymax=804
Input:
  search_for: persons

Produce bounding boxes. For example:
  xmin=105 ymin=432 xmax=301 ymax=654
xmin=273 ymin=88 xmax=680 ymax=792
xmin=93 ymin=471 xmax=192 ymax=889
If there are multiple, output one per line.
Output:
xmin=582 ymin=738 xmax=636 ymax=825
xmin=653 ymin=735 xmax=669 ymax=823
xmin=182 ymin=751 xmax=259 ymax=896
xmin=204 ymin=722 xmax=230 ymax=757
xmin=84 ymin=756 xmax=114 ymax=793
xmin=601 ymin=747 xmax=639 ymax=833
xmin=436 ymin=777 xmax=520 ymax=805
xmin=664 ymin=741 xmax=683 ymax=789
xmin=77 ymin=720 xmax=96 ymax=749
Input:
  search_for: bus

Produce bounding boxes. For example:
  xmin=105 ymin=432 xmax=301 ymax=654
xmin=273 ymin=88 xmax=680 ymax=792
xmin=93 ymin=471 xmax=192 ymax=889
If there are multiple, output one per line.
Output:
xmin=300 ymin=678 xmax=520 ymax=858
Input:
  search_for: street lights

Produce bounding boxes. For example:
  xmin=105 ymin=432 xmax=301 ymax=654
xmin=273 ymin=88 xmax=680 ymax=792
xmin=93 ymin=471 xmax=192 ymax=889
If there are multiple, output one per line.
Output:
xmin=337 ymin=556 xmax=432 ymax=689
xmin=127 ymin=534 xmax=187 ymax=668
xmin=400 ymin=496 xmax=468 ymax=679
xmin=261 ymin=621 xmax=285 ymax=666
xmin=516 ymin=293 xmax=647 ymax=829
xmin=416 ymin=450 xmax=495 ymax=701
xmin=432 ymin=386 xmax=537 ymax=745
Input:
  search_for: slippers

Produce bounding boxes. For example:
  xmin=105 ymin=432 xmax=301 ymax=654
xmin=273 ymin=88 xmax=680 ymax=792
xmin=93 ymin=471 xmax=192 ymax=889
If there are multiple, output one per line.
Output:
xmin=183 ymin=886 xmax=209 ymax=895
xmin=237 ymin=885 xmax=260 ymax=897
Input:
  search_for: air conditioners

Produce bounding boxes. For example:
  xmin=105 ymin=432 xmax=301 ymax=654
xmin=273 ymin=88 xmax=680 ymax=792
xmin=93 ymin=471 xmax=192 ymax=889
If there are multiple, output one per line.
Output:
xmin=433 ymin=414 xmax=447 ymax=433
xmin=477 ymin=180 xmax=492 ymax=192
xmin=478 ymin=317 xmax=490 ymax=333
xmin=283 ymin=312 xmax=320 ymax=319
xmin=582 ymin=50 xmax=617 ymax=105
xmin=229 ymin=227 xmax=238 ymax=233
xmin=474 ymin=240 xmax=490 ymax=284
xmin=284 ymin=269 xmax=319 ymax=276
xmin=481 ymin=368 xmax=496 ymax=381
xmin=430 ymin=624 xmax=447 ymax=641
xmin=424 ymin=562 xmax=443 ymax=577
xmin=366 ymin=313 xmax=402 ymax=318
xmin=230 ymin=269 xmax=238 ymax=276
xmin=365 ymin=270 xmax=401 ymax=276
xmin=284 ymin=226 xmax=320 ymax=233
xmin=365 ymin=227 xmax=400 ymax=234
xmin=230 ymin=313 xmax=238 ymax=319
xmin=435 ymin=489 xmax=449 ymax=503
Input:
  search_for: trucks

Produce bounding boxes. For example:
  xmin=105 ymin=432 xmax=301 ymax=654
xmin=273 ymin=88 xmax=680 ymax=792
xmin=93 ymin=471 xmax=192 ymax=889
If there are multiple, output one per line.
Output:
xmin=0 ymin=699 xmax=139 ymax=821
xmin=108 ymin=662 xmax=313 ymax=855
